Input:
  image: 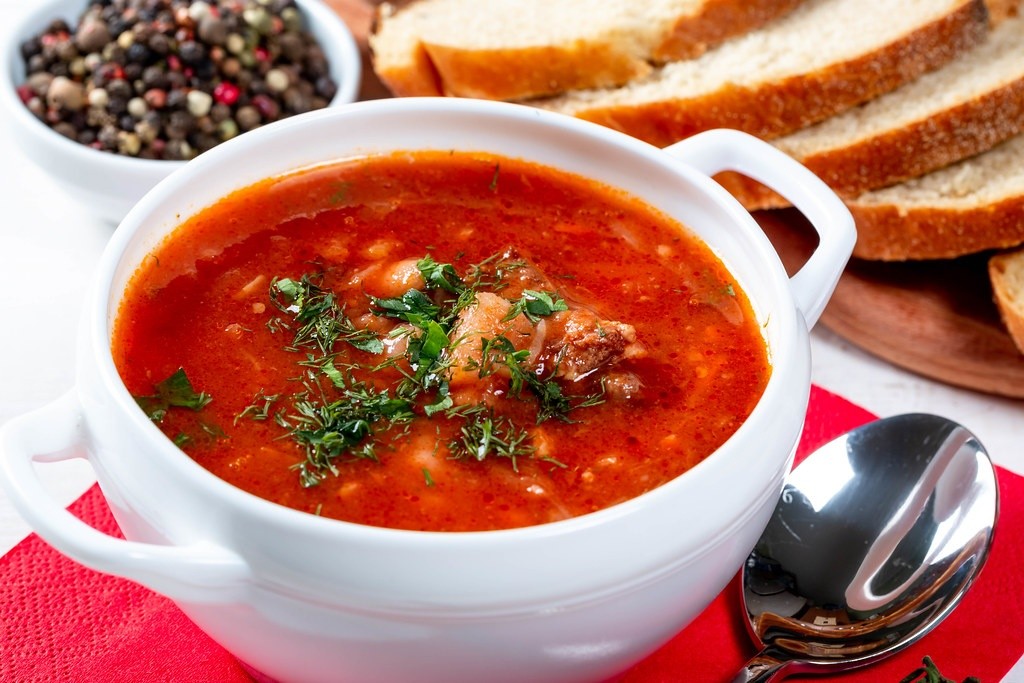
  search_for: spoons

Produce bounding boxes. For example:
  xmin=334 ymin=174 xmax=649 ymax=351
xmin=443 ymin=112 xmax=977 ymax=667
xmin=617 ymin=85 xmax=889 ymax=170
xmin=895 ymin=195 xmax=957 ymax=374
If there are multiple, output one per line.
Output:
xmin=727 ymin=414 xmax=998 ymax=683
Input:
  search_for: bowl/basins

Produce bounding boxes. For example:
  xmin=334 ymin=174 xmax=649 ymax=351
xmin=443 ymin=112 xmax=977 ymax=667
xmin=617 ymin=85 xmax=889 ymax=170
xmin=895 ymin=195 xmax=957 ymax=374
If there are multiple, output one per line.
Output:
xmin=0 ymin=0 xmax=363 ymax=225
xmin=0 ymin=96 xmax=857 ymax=683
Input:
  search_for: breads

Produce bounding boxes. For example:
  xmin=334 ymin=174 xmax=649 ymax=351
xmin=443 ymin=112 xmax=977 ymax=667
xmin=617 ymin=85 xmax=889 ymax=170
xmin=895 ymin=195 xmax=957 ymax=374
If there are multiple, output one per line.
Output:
xmin=369 ymin=1 xmax=1024 ymax=410
